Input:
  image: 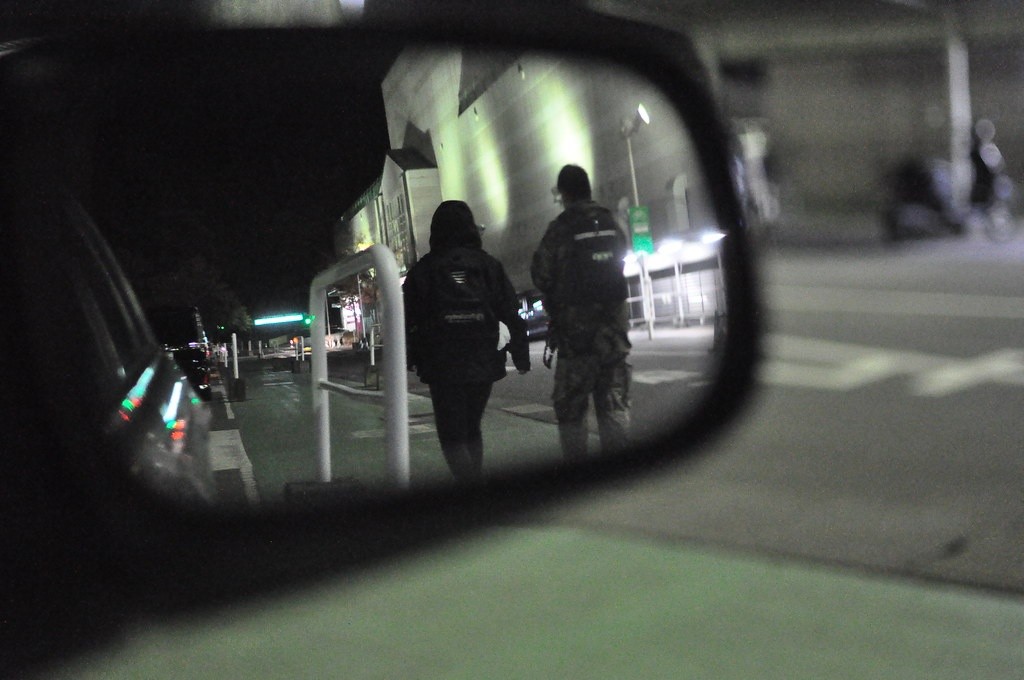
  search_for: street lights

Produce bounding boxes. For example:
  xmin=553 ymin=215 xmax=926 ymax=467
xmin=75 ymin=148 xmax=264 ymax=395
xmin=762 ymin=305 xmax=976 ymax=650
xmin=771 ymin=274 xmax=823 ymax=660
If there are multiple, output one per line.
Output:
xmin=625 ymin=103 xmax=654 ymax=339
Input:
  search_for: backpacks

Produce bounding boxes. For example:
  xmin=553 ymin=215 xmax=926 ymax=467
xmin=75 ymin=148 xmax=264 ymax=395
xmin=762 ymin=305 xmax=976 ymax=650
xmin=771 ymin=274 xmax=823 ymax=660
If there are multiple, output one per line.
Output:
xmin=538 ymin=256 xmax=606 ymax=366
xmin=414 ymin=252 xmax=502 ymax=382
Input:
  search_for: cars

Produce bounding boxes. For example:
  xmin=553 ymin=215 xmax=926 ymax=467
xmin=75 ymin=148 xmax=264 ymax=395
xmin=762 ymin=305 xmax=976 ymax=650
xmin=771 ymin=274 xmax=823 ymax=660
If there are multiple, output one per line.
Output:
xmin=292 ymin=328 xmax=348 ymax=352
xmin=515 ymin=289 xmax=550 ymax=339
xmin=166 ymin=349 xmax=211 ymax=399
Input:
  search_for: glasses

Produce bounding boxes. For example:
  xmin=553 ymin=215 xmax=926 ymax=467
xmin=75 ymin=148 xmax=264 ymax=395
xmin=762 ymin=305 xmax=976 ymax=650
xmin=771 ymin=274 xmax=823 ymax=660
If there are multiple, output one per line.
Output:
xmin=551 ymin=188 xmax=561 ymax=203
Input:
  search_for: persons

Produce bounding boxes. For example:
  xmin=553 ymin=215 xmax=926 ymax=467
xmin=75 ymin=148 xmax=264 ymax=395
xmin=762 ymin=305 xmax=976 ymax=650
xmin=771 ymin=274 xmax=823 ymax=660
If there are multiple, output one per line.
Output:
xmin=532 ymin=163 xmax=630 ymax=461
xmin=217 ymin=343 xmax=225 ymax=379
xmin=406 ymin=199 xmax=530 ymax=479
